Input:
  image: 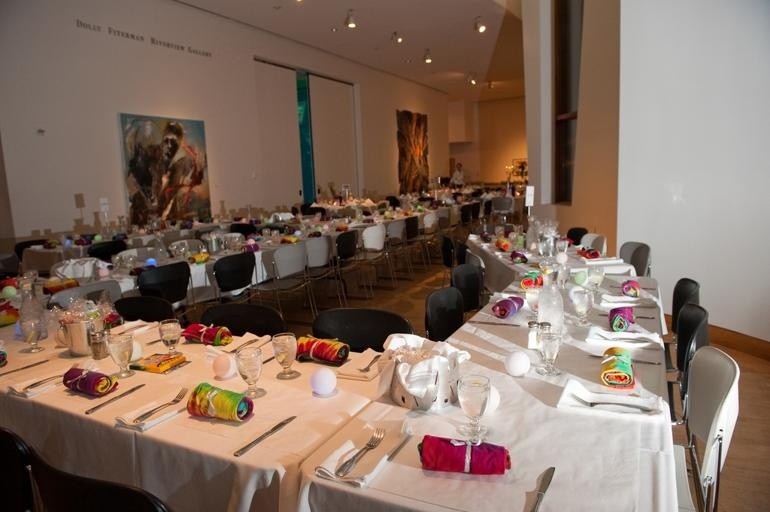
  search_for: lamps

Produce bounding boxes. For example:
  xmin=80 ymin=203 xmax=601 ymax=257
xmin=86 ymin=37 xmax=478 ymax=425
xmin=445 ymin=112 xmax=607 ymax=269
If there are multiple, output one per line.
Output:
xmin=345 ymin=9 xmax=494 ymax=89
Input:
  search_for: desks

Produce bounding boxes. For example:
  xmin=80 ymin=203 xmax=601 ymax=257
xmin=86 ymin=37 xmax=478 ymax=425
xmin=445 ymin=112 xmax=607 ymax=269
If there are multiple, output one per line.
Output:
xmin=466 ymin=229 xmax=637 ymax=296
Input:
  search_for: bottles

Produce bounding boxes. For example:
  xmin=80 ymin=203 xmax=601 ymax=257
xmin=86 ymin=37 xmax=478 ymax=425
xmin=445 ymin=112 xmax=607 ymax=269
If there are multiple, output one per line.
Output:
xmin=88 ymin=328 xmax=112 ymax=361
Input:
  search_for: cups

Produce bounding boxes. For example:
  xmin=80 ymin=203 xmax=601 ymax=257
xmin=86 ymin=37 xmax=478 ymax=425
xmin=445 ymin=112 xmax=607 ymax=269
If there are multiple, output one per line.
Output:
xmin=57 ymin=322 xmax=92 ymax=357
xmin=317 ymin=196 xmax=399 ymax=229
xmin=106 ymin=209 xmax=280 ymax=275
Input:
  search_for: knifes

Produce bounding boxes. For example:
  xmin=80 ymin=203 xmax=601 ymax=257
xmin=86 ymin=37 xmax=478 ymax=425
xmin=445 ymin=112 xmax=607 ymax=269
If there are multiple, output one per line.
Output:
xmin=232 ymin=413 xmax=300 ymax=458
xmin=85 ymin=380 xmax=149 ymax=416
xmin=0 ymin=358 xmax=51 ymax=377
xmin=527 ymin=465 xmax=558 ymax=512
xmin=462 ymin=318 xmax=522 ymax=327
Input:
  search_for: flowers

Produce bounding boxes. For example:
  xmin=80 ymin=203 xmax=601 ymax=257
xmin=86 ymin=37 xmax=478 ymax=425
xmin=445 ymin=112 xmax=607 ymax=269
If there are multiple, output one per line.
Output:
xmin=504 ymin=165 xmax=516 ymax=182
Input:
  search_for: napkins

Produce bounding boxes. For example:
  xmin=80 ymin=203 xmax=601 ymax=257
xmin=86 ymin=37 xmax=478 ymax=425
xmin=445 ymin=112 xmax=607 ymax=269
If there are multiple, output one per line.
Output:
xmin=8 ymin=360 xmax=100 ymax=400
xmin=107 ymin=319 xmax=159 ymax=341
xmin=599 ymin=294 xmax=658 ymax=311
xmin=556 ymin=378 xmax=663 ymax=421
xmin=203 ymin=331 xmax=273 ymax=358
xmin=585 ymin=325 xmax=662 ymax=352
xmin=114 ymin=388 xmax=196 ymax=433
xmin=333 ymin=347 xmax=391 ymax=382
xmin=314 ymin=416 xmax=414 ymax=487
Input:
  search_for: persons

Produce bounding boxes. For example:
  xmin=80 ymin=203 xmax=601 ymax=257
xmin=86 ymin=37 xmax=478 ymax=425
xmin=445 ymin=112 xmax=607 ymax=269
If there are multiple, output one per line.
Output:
xmin=450 ymin=163 xmax=464 ymax=188
xmin=159 ymin=122 xmax=196 ymax=223
xmin=127 ymin=143 xmax=170 ymax=227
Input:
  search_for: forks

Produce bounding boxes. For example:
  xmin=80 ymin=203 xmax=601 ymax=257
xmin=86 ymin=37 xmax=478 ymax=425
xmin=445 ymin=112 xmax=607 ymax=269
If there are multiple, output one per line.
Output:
xmin=132 ymin=384 xmax=189 ymax=423
xmin=220 ymin=336 xmax=258 ymax=354
xmin=356 ymin=354 xmax=382 ymax=376
xmin=570 ymin=392 xmax=654 ymax=413
xmin=336 ymin=428 xmax=386 ymax=480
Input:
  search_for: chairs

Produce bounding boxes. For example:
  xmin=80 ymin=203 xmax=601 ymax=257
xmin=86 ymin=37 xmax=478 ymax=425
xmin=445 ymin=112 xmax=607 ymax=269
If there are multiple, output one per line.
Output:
xmin=662 ymin=277 xmax=710 ymax=424
xmin=113 ymin=296 xmax=177 ymax=322
xmin=426 ymin=288 xmax=466 ymax=341
xmin=567 ymin=226 xmax=608 ymax=256
xmin=676 ymin=345 xmax=741 ymax=512
xmin=200 ymin=303 xmax=283 ymax=336
xmin=619 ymin=241 xmax=652 ymax=277
xmin=312 ymin=309 xmax=412 ymax=350
xmin=1 ymin=183 xmax=526 ymax=309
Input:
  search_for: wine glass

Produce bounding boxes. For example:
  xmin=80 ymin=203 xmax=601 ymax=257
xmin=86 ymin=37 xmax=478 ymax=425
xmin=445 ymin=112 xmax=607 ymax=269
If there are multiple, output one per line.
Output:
xmin=19 ymin=270 xmax=49 ymax=354
xmin=520 ymin=214 xmax=603 ymax=374
xmin=453 ymin=373 xmax=492 ymax=436
xmin=158 ymin=319 xmax=182 ymax=355
xmin=269 ymin=332 xmax=303 ymax=380
xmin=234 ymin=345 xmax=268 ymax=399
xmin=105 ymin=334 xmax=136 ymax=378
